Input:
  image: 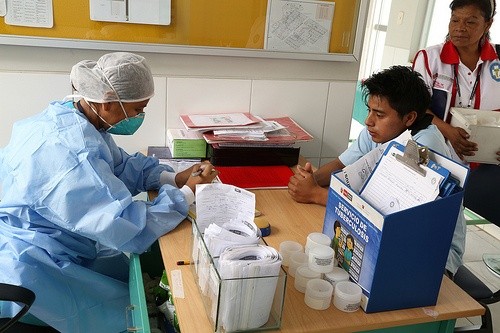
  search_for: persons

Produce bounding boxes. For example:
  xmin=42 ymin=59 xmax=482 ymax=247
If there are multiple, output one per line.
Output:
xmin=288 ymin=65 xmax=467 ymax=282
xmin=0 ymin=52 xmax=219 ymax=333
xmin=412 ymin=0 xmax=500 ymax=228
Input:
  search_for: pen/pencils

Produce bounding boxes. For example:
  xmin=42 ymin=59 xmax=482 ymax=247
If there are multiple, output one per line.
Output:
xmin=192 ymin=167 xmax=204 ymax=177
xmin=177 ymin=261 xmax=190 ymax=265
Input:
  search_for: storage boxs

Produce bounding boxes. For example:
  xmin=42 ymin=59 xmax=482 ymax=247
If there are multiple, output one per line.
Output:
xmin=166 ymin=126 xmax=301 ymax=167
xmin=190 ymin=219 xmax=288 ymax=333
xmin=319 ymin=143 xmax=470 ymax=317
xmin=447 ymin=107 xmax=500 ymax=167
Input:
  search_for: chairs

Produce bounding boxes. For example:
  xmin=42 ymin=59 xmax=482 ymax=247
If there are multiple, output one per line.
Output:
xmin=444 ymin=162 xmax=500 ymax=333
xmin=0 ymin=283 xmax=60 ymax=333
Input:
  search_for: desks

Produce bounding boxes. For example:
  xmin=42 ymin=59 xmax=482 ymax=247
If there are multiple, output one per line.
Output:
xmin=125 ymin=146 xmax=486 ymax=333
